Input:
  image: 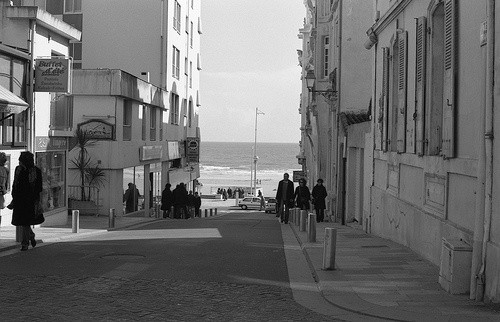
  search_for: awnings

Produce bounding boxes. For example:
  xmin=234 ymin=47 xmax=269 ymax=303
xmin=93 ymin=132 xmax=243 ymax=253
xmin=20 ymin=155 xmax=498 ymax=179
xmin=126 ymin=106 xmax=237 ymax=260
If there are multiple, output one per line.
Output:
xmin=0 ymin=86 xmax=29 ymax=125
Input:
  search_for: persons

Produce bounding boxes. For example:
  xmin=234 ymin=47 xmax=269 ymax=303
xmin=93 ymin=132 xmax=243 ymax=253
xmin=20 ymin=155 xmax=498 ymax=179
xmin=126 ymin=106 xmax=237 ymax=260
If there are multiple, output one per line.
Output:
xmin=7 ymin=152 xmax=45 ymax=251
xmin=217 ymin=187 xmax=244 ymax=201
xmin=312 ymin=179 xmax=327 ymax=223
xmin=275 ymin=173 xmax=294 ymax=224
xmin=0 ymin=152 xmax=10 ymax=209
xmin=160 ymin=183 xmax=201 ymax=219
xmin=257 ymin=190 xmax=266 ymax=211
xmin=290 ymin=178 xmax=311 ymax=211
xmin=123 ymin=183 xmax=141 ymax=213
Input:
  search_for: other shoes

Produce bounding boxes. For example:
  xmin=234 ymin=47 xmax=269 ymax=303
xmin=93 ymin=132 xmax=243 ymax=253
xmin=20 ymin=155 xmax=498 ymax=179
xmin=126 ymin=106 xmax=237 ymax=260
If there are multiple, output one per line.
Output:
xmin=22 ymin=246 xmax=28 ymax=250
xmin=31 ymin=237 xmax=36 ymax=247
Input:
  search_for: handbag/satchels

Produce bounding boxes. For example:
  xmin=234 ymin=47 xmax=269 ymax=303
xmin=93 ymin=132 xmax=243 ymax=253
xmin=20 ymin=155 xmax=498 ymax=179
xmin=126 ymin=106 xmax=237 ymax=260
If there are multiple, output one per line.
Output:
xmin=312 ymin=199 xmax=315 ymax=205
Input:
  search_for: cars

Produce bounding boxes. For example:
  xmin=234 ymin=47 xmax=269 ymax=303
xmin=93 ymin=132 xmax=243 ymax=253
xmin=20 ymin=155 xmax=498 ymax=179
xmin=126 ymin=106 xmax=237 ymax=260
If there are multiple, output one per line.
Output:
xmin=238 ymin=196 xmax=268 ymax=210
xmin=265 ymin=198 xmax=277 ymax=214
